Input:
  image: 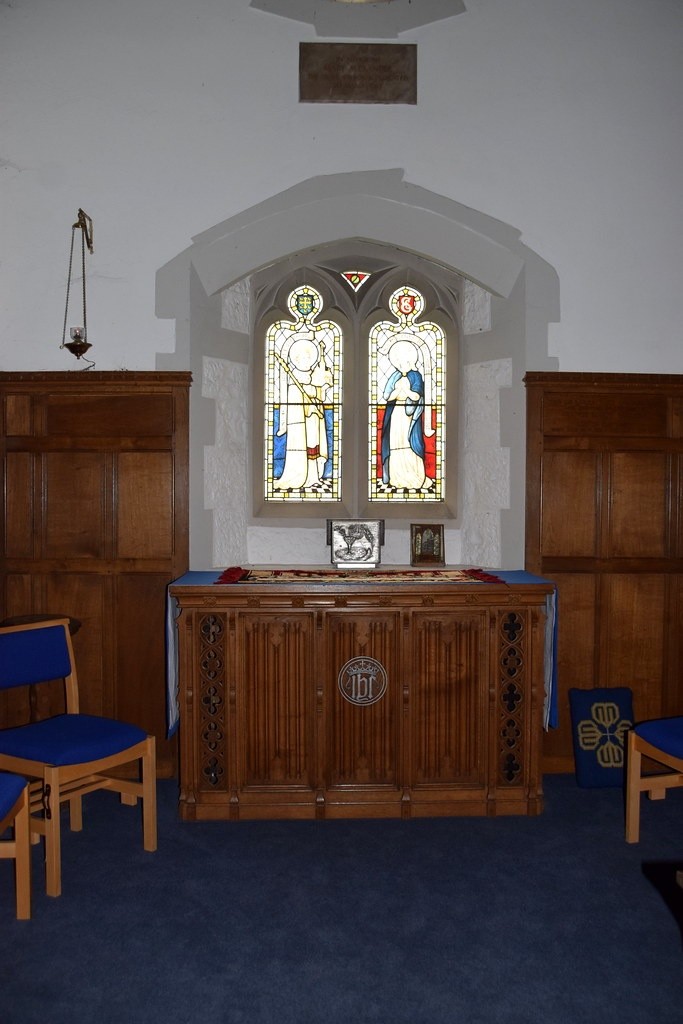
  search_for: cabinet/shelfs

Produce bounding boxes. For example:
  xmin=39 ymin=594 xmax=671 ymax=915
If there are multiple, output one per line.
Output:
xmin=1 ymin=371 xmax=189 ymax=780
xmin=166 ymin=571 xmax=555 ymax=822
xmin=523 ymin=370 xmax=679 ymax=775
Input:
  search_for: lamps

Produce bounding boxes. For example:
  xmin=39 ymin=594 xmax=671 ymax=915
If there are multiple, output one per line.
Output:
xmin=60 ymin=208 xmax=96 ymax=357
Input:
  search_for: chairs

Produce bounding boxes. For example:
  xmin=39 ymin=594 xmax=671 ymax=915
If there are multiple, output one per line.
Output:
xmin=1 ymin=618 xmax=156 ymax=920
xmin=626 ymin=717 xmax=683 ymax=844
